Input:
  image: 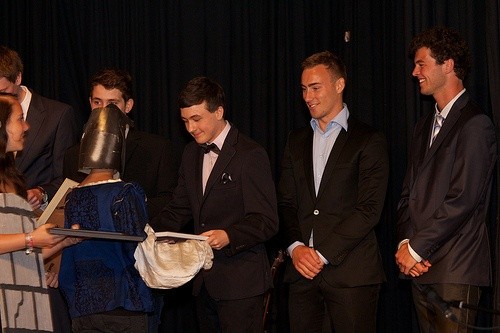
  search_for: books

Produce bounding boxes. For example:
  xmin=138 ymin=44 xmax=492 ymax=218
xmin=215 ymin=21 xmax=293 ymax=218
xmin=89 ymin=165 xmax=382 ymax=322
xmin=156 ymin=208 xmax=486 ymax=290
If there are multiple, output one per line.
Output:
xmin=155 ymin=232 xmax=212 ymax=241
xmin=50 ymin=229 xmax=146 ymax=243
xmin=34 ymin=178 xmax=82 ymax=231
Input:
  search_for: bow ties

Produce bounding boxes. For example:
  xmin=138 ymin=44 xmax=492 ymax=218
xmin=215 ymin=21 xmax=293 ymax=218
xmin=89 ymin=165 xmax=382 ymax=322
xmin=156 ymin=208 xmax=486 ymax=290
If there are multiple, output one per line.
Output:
xmin=200 ymin=143 xmax=222 ymax=156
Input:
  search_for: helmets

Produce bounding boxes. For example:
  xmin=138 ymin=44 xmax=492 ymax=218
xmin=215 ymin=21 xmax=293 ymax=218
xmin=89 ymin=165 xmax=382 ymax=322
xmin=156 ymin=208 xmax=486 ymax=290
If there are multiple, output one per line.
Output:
xmin=78 ymin=105 xmax=134 ymax=179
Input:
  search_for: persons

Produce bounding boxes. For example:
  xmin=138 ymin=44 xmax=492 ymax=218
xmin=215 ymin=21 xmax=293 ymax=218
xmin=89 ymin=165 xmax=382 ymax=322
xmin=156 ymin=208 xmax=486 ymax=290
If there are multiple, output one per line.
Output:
xmin=57 ymin=103 xmax=171 ymax=333
xmin=394 ymin=27 xmax=500 ymax=333
xmin=280 ymin=50 xmax=389 ymax=333
xmin=0 ymin=45 xmax=81 ymax=212
xmin=0 ymin=91 xmax=66 ymax=333
xmin=150 ymin=75 xmax=278 ymax=333
xmin=88 ymin=62 xmax=166 ymax=222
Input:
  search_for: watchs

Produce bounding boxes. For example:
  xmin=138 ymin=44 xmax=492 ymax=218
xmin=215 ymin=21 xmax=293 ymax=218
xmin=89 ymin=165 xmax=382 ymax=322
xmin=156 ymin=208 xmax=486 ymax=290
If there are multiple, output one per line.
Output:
xmin=23 ymin=230 xmax=34 ymax=256
xmin=35 ymin=185 xmax=50 ymax=206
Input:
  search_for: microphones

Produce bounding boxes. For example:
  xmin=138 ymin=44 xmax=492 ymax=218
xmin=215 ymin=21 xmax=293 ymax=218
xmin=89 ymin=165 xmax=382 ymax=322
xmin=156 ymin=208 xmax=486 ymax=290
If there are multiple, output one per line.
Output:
xmin=423 ymin=286 xmax=457 ymax=322
